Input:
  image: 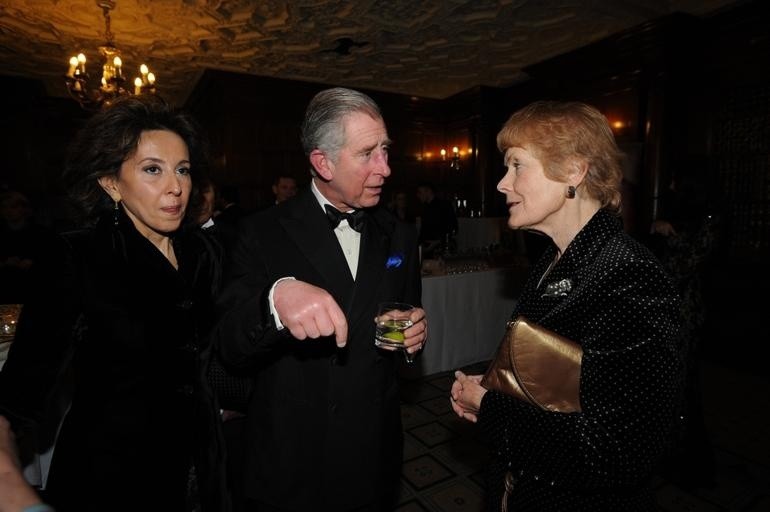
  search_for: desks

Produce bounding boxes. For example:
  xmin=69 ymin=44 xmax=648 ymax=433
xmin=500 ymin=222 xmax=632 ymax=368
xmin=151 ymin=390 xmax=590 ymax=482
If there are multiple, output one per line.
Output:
xmin=401 ymin=266 xmax=521 ymax=378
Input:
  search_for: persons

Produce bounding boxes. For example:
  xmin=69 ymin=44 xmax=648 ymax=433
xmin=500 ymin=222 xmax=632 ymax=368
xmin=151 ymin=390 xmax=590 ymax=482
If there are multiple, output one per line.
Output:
xmin=212 ymin=181 xmax=247 ymax=221
xmin=448 ymin=101 xmax=691 ymax=509
xmin=183 ymin=171 xmax=223 ymax=240
xmin=270 ymin=171 xmax=302 ymax=205
xmin=1 ymin=100 xmax=231 ymax=512
xmin=389 ymin=189 xmax=422 ymax=238
xmin=0 ymin=417 xmax=53 ymax=512
xmin=416 ymin=177 xmax=458 ymax=255
xmin=216 ymin=89 xmax=426 ymax=511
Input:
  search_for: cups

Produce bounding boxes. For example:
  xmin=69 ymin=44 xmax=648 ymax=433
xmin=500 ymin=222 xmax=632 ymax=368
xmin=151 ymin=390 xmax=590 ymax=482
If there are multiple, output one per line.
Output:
xmin=374 ymin=302 xmax=417 ymax=351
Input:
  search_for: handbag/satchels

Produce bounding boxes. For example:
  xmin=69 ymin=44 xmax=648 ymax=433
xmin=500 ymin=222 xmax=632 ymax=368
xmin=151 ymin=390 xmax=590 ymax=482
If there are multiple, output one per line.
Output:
xmin=205 ymin=356 xmax=255 ymax=414
xmin=480 ymin=314 xmax=592 ymax=415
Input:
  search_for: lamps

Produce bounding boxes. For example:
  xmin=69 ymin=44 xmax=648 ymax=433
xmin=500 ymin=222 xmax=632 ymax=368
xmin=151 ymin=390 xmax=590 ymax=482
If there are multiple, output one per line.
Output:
xmin=65 ymin=0 xmax=157 ymax=116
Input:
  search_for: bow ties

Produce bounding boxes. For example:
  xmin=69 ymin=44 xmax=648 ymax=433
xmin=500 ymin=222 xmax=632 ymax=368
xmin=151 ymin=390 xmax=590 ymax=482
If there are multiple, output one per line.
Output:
xmin=323 ymin=201 xmax=369 ymax=233
